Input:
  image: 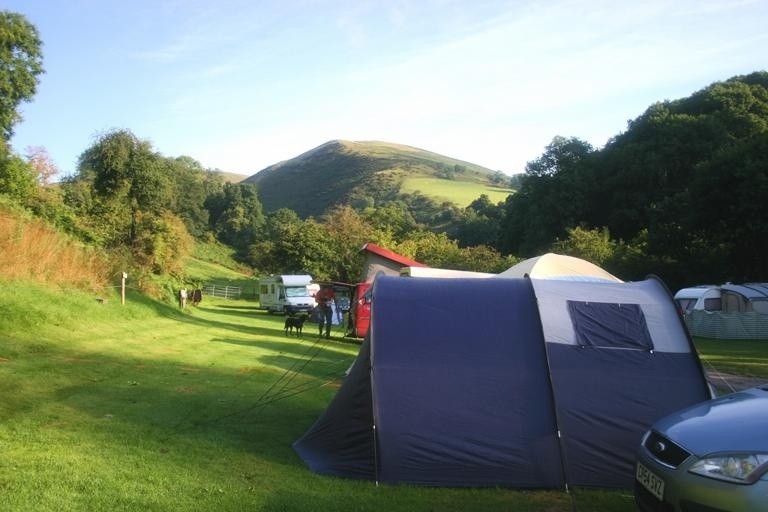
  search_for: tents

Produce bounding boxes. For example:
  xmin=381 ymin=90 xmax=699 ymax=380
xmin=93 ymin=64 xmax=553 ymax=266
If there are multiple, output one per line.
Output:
xmin=290 ymin=268 xmax=715 ymax=490
xmin=489 ymin=250 xmax=628 ymax=283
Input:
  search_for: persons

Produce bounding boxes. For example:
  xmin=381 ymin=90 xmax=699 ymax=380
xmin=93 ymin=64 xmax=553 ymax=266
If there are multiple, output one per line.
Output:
xmin=315 ymin=286 xmax=335 ymax=338
xmin=178 ymin=287 xmax=188 ymax=309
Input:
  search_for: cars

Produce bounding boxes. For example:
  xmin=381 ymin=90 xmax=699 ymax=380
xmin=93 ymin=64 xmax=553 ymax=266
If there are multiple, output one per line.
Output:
xmin=632 ymin=384 xmax=767 ymax=511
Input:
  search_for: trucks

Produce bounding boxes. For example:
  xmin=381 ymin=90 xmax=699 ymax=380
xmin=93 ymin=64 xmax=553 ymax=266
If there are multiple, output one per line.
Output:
xmin=259 ymin=274 xmax=317 ymax=316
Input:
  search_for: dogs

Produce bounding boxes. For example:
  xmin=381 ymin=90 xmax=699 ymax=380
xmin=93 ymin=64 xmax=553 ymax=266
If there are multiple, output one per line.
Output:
xmin=284 ymin=314 xmax=309 ymax=338
